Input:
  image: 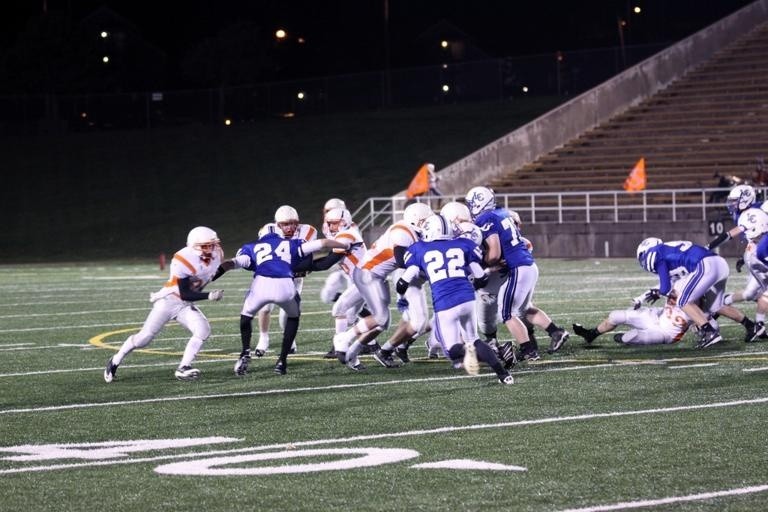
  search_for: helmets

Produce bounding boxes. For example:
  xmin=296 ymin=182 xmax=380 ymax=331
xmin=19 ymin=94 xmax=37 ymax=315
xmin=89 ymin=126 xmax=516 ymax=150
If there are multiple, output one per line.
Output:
xmin=421 ymin=215 xmax=452 ymax=242
xmin=275 ymin=205 xmax=299 ymax=239
xmin=453 ymin=222 xmax=482 ymax=246
xmin=403 ymin=203 xmax=434 ymax=233
xmin=500 ymin=207 xmax=521 ymax=226
xmin=637 ymin=237 xmax=663 ymax=273
xmin=258 ymin=223 xmax=285 ymax=240
xmin=726 ymin=185 xmax=756 ymax=214
xmin=440 ymin=201 xmax=472 ymax=235
xmin=738 ymin=208 xmax=768 ymax=239
xmin=326 ymin=208 xmax=352 ymax=229
xmin=465 ymin=186 xmax=496 ymax=220
xmin=187 ymin=226 xmax=220 ymax=259
xmin=761 ymin=200 xmax=768 ymax=212
xmin=323 ymin=198 xmax=346 ymax=214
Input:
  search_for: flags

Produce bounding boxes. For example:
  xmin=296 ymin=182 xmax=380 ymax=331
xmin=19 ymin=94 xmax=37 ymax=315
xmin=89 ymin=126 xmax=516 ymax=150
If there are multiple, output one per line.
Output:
xmin=406 ymin=163 xmax=429 ymax=199
xmin=623 ymin=157 xmax=647 ymax=191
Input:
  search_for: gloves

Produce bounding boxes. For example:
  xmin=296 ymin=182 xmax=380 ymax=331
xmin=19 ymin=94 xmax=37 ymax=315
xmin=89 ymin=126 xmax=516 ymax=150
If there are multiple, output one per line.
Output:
xmin=208 ymin=289 xmax=224 ymax=301
xmin=736 ymin=257 xmax=745 ymax=272
xmin=645 ymin=289 xmax=661 ymax=306
xmin=396 ymin=296 xmax=408 ymax=313
xmin=345 ymin=242 xmax=363 ymax=253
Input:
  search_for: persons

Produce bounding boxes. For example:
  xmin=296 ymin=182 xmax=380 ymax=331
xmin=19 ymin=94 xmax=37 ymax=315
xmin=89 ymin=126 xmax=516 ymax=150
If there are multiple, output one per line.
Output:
xmin=296 ymin=209 xmax=367 ymax=360
xmin=427 ymin=163 xmax=443 ymax=210
xmin=372 ymin=267 xmax=433 ymax=369
xmin=323 ymin=199 xmax=370 ymax=318
xmin=396 ymin=214 xmax=515 ymax=386
xmin=465 ymin=186 xmax=570 ymax=362
xmin=254 ymin=204 xmax=317 ymax=357
xmin=104 ymin=226 xmax=224 ymax=383
xmin=474 ymin=209 xmax=533 ymax=350
xmin=703 ymin=183 xmax=768 ymax=250
xmin=211 ymin=223 xmax=364 ymax=376
xmin=425 ymin=201 xmax=475 ymax=359
xmin=571 ymin=265 xmax=707 ymax=345
xmin=714 ymin=200 xmax=768 ymax=340
xmin=333 ymin=199 xmax=433 ymax=372
xmin=636 ymin=237 xmax=766 ymax=351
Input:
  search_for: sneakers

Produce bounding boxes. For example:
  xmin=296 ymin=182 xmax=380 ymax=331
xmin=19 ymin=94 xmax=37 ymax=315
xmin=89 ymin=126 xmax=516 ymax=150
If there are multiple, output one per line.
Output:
xmin=614 ymin=333 xmax=624 ymax=344
xmin=425 ymin=339 xmax=430 ymax=348
xmin=234 ymin=349 xmax=251 ymax=376
xmin=497 ymin=375 xmax=514 ymax=385
xmin=274 ymin=361 xmax=286 ymax=375
xmin=333 ymin=333 xmax=347 ymax=363
xmin=255 ymin=337 xmax=269 ymax=357
xmin=515 ymin=350 xmax=541 ymax=362
xmin=345 ymin=359 xmax=367 ymax=372
xmin=463 ymin=342 xmax=480 ymax=378
xmin=529 ymin=341 xmax=539 ymax=351
xmin=695 ymin=331 xmax=722 ymax=350
xmin=368 ymin=342 xmax=381 ymax=353
xmin=753 ymin=321 xmax=768 ymax=340
xmin=428 ymin=349 xmax=439 ymax=359
xmin=745 ymin=322 xmax=765 ymax=343
xmin=374 ymin=348 xmax=394 ymax=368
xmin=395 ymin=344 xmax=410 ymax=365
xmin=324 ymin=346 xmax=338 ymax=359
xmin=288 ymin=339 xmax=297 ymax=354
xmin=175 ymin=365 xmax=201 ymax=380
xmin=573 ymin=324 xmax=594 ymax=343
xmin=711 ymin=293 xmax=731 ymax=320
xmin=543 ymin=328 xmax=569 ymax=355
xmin=104 ymin=357 xmax=118 ymax=383
